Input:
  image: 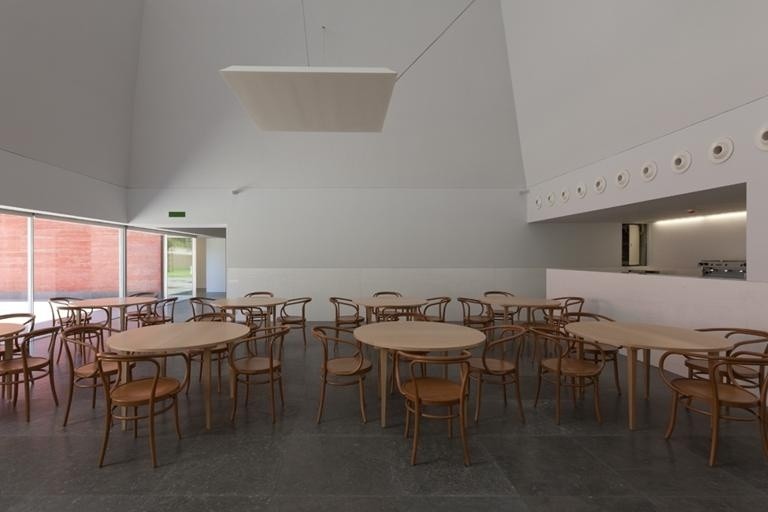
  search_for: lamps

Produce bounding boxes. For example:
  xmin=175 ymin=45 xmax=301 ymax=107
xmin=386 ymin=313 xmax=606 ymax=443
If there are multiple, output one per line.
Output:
xmin=219 ymin=0 xmax=475 ymax=133
